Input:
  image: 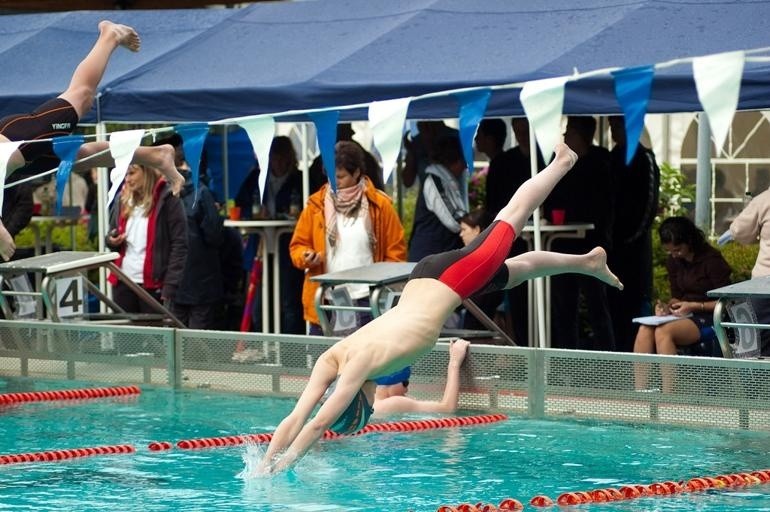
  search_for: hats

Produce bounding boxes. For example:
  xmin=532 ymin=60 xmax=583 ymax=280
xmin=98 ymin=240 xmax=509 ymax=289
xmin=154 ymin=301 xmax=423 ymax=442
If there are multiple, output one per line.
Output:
xmin=373 ymin=364 xmax=411 ymax=385
xmin=321 ymin=377 xmax=372 ymax=435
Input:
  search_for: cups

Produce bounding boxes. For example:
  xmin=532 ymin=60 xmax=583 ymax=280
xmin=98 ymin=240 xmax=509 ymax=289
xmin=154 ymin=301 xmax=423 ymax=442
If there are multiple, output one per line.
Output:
xmin=227 ymin=206 xmax=241 ymax=220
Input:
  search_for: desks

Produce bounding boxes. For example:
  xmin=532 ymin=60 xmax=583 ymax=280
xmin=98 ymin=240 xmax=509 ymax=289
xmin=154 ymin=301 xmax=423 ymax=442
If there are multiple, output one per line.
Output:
xmin=521 ymin=223 xmax=594 ymax=350
xmin=26 ymin=213 xmax=82 ymax=321
xmin=223 ymin=219 xmax=298 ymax=364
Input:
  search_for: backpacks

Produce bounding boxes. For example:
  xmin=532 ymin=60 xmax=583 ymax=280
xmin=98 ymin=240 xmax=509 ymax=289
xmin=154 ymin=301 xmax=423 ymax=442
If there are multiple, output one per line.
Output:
xmin=211 ymin=210 xmax=246 ymax=305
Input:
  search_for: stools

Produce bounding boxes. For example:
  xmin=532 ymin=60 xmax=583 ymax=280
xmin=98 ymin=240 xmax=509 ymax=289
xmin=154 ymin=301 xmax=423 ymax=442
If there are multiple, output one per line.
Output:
xmin=676 ymin=339 xmax=720 ymax=356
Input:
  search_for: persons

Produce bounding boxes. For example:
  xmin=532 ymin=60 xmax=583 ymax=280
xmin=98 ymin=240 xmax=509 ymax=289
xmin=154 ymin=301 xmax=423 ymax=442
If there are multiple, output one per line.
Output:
xmin=633 ymin=216 xmax=740 ymax=405
xmin=728 ymin=187 xmax=770 ymax=359
xmin=371 ymin=340 xmax=471 ymax=423
xmin=0 ymin=219 xmax=16 ymax=262
xmin=83 ymin=113 xmax=660 ymax=388
xmin=257 ymin=143 xmax=624 ymax=482
xmin=0 ymin=18 xmax=185 ymax=242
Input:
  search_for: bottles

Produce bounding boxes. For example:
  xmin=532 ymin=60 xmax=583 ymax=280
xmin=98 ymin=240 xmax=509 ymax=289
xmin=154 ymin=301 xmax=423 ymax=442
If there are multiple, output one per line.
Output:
xmin=250 ymin=190 xmax=298 ymax=221
